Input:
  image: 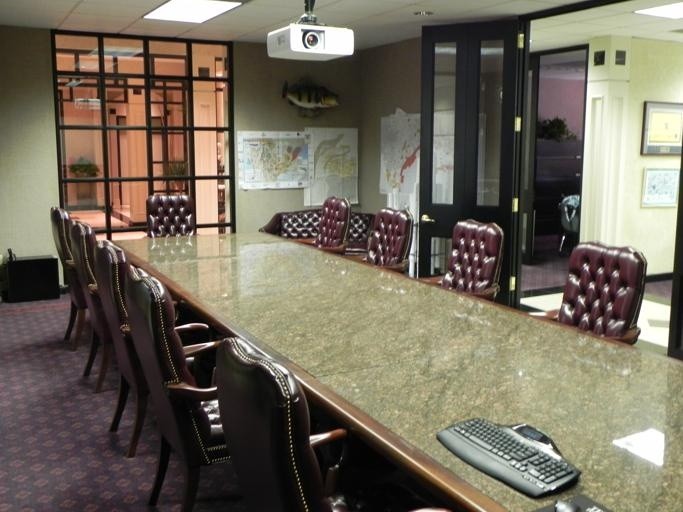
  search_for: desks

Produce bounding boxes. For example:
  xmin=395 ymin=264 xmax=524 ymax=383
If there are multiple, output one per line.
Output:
xmin=110 ymin=230 xmax=683 ymax=512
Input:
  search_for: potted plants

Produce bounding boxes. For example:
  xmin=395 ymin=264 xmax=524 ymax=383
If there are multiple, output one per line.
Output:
xmin=68 ymin=157 xmax=100 ymax=198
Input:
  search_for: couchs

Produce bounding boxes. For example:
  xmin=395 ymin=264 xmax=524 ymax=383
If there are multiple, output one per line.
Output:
xmin=259 ymin=208 xmax=376 ymax=256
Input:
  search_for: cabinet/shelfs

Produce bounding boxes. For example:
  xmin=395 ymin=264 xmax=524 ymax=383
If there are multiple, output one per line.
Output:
xmin=7 ymin=255 xmax=60 ymax=302
xmin=534 ymin=140 xmax=579 ymax=235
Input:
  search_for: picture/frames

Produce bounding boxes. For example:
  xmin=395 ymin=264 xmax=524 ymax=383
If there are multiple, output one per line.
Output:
xmin=641 ymin=100 xmax=683 ymax=155
xmin=641 ymin=167 xmax=680 ymax=207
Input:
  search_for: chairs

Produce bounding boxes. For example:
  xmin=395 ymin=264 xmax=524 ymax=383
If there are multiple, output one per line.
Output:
xmin=146 ymin=195 xmax=196 ymax=238
xmin=342 ymin=208 xmax=414 ymax=275
xmin=419 ymin=218 xmax=504 ymax=302
xmin=528 ymin=241 xmax=648 ymax=347
xmin=291 ymin=196 xmax=351 ymax=257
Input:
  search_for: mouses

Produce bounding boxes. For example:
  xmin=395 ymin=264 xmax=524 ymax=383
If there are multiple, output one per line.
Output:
xmin=554 ymin=498 xmax=580 ymax=512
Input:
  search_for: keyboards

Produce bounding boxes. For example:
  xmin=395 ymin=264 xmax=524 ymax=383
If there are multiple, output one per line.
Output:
xmin=435 ymin=417 xmax=581 ymax=497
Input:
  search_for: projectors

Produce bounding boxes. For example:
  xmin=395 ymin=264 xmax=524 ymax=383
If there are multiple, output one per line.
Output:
xmin=267 ymin=23 xmax=355 ymax=62
